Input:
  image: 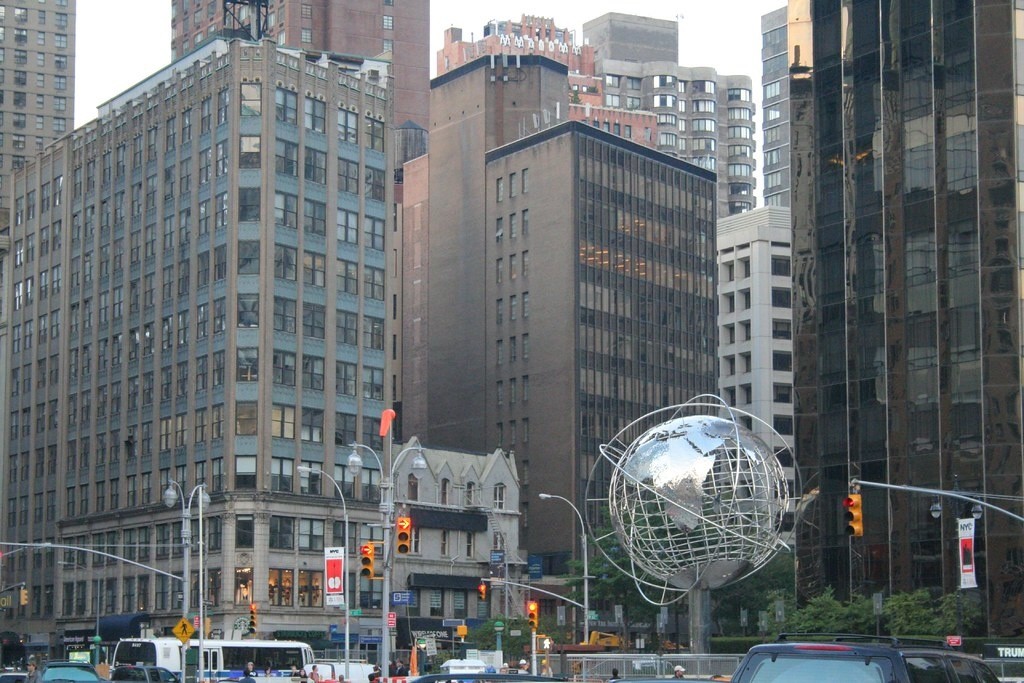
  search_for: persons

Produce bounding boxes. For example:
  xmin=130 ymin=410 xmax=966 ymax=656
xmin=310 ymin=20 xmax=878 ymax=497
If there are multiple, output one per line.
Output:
xmin=445 ymin=659 xmax=532 ymax=683
xmin=541 ymin=660 xmax=552 ymax=677
xmin=25 ymin=661 xmax=39 ymax=683
xmin=308 ymin=664 xmax=319 ymax=683
xmin=368 ymin=659 xmax=408 ymax=683
xmin=672 ymin=665 xmax=686 ymax=679
xmin=610 ymin=668 xmax=621 ymax=680
xmin=240 ymin=669 xmax=256 ymax=683
xmin=339 ymin=675 xmax=346 ymax=683
xmin=289 ymin=666 xmax=300 ymax=677
xmin=299 ymin=669 xmax=308 ymax=683
xmin=264 ymin=666 xmax=273 ymax=677
xmin=243 ymin=662 xmax=258 ymax=677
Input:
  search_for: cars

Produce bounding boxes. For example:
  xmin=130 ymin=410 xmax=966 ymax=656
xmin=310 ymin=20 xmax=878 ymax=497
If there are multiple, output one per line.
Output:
xmin=0 ymin=660 xmax=182 ymax=683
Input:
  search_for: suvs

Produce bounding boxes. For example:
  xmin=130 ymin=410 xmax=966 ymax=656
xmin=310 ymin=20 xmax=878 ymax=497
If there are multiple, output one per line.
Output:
xmin=729 ymin=632 xmax=1000 ymax=683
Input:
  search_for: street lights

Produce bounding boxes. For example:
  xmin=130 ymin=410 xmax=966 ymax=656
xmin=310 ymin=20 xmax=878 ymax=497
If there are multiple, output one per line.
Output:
xmin=494 ymin=622 xmax=504 ymax=651
xmin=416 ymin=639 xmax=426 ymax=674
xmin=296 ymin=466 xmax=350 ymax=678
xmin=93 ymin=634 xmax=102 ymax=666
xmin=539 ymin=493 xmax=597 ymax=644
xmin=163 ymin=479 xmax=211 ymax=683
xmin=347 ymin=442 xmax=427 ymax=678
xmin=57 ymin=561 xmax=100 ymax=666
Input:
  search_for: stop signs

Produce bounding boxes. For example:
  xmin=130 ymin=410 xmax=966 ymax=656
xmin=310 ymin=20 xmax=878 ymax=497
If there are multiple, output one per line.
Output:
xmin=195 ymin=621 xmax=199 ymax=625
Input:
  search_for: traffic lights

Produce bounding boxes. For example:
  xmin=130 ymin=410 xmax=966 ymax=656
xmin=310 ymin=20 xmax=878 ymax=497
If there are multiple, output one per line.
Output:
xmin=360 ymin=545 xmax=374 ymax=578
xmin=528 ymin=603 xmax=538 ymax=628
xmin=478 ymin=585 xmax=486 ymax=600
xmin=250 ymin=604 xmax=258 ymax=637
xmin=397 ymin=517 xmax=411 ymax=554
xmin=537 ymin=637 xmax=551 ymax=651
xmin=20 ymin=590 xmax=28 ymax=605
xmin=843 ymin=494 xmax=864 ymax=536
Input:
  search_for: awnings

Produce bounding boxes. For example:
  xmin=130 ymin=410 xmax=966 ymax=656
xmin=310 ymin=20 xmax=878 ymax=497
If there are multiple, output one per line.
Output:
xmin=396 ymin=617 xmax=454 ymax=650
xmin=94 ymin=613 xmax=150 ymax=642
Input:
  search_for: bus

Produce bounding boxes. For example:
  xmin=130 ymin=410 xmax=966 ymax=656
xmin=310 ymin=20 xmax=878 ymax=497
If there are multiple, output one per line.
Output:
xmin=113 ymin=638 xmax=315 ymax=682
